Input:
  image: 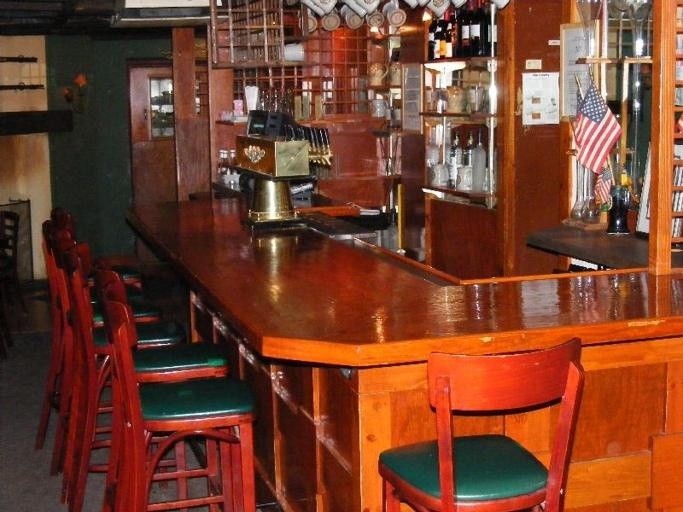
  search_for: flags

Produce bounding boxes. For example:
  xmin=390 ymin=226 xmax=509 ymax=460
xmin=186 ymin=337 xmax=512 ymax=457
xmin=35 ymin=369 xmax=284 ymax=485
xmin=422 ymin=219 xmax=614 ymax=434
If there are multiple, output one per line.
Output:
xmin=572 ymin=80 xmax=623 ymax=175
xmin=575 ymin=86 xmax=612 ymax=206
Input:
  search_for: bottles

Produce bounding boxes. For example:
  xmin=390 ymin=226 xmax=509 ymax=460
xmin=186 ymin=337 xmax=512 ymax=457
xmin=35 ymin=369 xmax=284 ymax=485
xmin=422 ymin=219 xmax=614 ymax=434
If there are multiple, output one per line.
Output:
xmin=451 ymin=130 xmax=462 ymax=163
xmin=216 ymin=149 xmax=241 ymax=185
xmin=471 ymin=127 xmax=486 ymax=194
xmin=257 ymin=88 xmax=290 ymax=112
xmin=464 ymin=131 xmax=475 ymax=166
xmin=428 ymin=0 xmax=497 ymax=60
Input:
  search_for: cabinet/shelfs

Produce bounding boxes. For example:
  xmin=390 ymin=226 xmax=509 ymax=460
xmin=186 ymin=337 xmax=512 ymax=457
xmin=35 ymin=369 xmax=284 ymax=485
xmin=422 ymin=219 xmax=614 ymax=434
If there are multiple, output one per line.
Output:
xmin=417 ymin=55 xmax=500 ymax=203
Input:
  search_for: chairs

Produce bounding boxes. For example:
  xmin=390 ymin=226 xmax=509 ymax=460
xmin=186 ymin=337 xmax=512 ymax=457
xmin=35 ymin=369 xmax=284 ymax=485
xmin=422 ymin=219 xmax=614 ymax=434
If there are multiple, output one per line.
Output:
xmin=376 ymin=334 xmax=586 ymax=511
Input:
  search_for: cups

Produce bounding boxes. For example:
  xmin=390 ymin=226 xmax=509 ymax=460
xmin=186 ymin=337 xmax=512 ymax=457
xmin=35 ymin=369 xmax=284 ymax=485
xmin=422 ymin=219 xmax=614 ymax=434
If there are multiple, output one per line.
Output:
xmin=430 ymin=165 xmax=449 ymax=187
xmin=371 ymin=100 xmax=389 ymax=117
xmin=456 ymin=167 xmax=473 ymax=191
xmin=606 ymin=185 xmax=631 ymax=235
